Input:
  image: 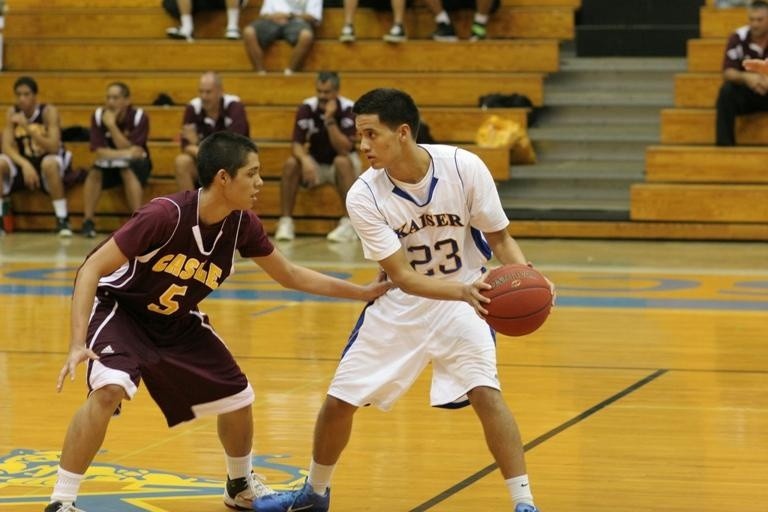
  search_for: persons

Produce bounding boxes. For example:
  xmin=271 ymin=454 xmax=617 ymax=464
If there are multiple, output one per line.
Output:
xmin=43 ymin=127 xmax=393 ymax=512
xmin=0 ymin=76 xmax=73 ymax=234
xmin=715 ymin=0 xmax=767 ymax=148
xmin=79 ymin=83 xmax=152 ymax=234
xmin=274 ymin=72 xmax=360 ymax=242
xmin=252 ymin=88 xmax=557 ymax=512
xmin=162 ymin=0 xmax=498 ymax=74
xmin=175 ymin=71 xmax=249 ymax=189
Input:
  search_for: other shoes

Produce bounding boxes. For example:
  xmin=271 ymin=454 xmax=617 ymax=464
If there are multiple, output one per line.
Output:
xmin=383 ymin=26 xmax=408 ymax=42
xmin=167 ymin=26 xmax=192 ymax=39
xmin=224 ymin=26 xmax=241 ymax=38
xmin=471 ymin=25 xmax=486 ymax=42
xmin=340 ymin=26 xmax=356 ymax=41
xmin=433 ymin=24 xmax=459 ymax=43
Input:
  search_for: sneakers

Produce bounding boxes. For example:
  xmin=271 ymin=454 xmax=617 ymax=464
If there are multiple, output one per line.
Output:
xmin=275 ymin=216 xmax=295 ymax=241
xmin=55 ymin=215 xmax=97 ymax=238
xmin=223 ymin=470 xmax=330 ymax=512
xmin=326 ymin=216 xmax=358 ymax=242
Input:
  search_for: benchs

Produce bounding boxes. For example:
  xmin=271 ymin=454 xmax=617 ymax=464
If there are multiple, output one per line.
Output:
xmin=1 ymin=0 xmax=582 ymax=231
xmin=628 ymin=1 xmax=767 ymax=229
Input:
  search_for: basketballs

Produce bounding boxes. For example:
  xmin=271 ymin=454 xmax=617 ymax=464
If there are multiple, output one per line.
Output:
xmin=478 ymin=263 xmax=551 ymax=336
